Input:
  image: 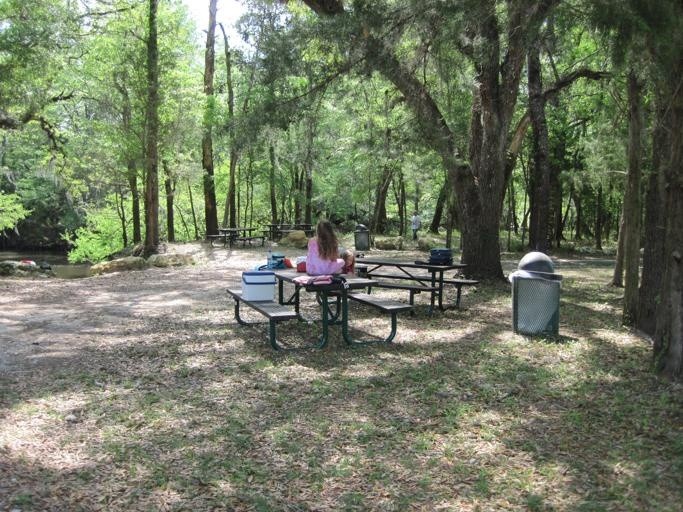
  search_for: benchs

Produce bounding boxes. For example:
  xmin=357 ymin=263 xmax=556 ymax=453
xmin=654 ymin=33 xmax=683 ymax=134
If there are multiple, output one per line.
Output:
xmin=227 ymin=272 xmax=482 ymax=358
xmin=206 ymin=230 xmax=316 ymax=249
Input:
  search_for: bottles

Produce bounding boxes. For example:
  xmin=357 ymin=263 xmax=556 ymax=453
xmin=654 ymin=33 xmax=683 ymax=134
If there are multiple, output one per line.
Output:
xmin=266 ymin=249 xmax=273 ymax=269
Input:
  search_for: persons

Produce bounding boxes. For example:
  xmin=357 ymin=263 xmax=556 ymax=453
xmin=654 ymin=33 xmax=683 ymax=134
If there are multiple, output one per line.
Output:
xmin=305 ymin=220 xmax=347 ymax=276
xmin=410 ymin=210 xmax=421 ymax=241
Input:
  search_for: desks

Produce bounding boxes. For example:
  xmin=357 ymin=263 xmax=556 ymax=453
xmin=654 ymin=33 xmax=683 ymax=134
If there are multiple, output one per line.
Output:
xmin=218 ymin=224 xmax=313 ymax=250
xmin=354 ymin=258 xmax=469 ymax=318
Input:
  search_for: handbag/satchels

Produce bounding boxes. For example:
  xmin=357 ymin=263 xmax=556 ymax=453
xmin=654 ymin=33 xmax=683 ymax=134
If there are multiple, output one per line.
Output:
xmin=428 ymin=248 xmax=452 ymax=265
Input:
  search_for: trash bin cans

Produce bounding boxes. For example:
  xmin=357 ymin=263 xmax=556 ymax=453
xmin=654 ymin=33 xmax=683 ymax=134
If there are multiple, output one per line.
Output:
xmin=354 ymin=224 xmax=370 ymax=251
xmin=512 ymin=251 xmax=563 ymax=336
